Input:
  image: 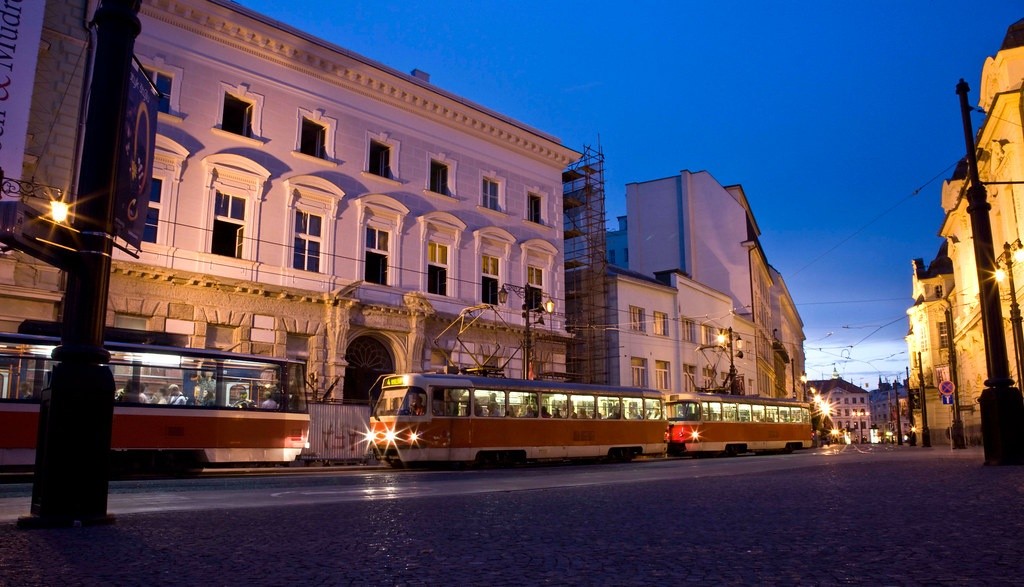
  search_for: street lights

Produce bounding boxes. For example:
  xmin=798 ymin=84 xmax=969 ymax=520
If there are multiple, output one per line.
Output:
xmin=498 ymin=282 xmax=555 ymax=381
xmin=718 ymin=327 xmax=744 ymax=396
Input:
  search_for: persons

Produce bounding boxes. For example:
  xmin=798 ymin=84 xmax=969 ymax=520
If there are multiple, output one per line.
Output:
xmin=115 ymin=359 xmax=282 ymax=409
xmin=18 ymin=381 xmax=31 ymax=399
xmin=404 ymin=394 xmax=662 ymax=420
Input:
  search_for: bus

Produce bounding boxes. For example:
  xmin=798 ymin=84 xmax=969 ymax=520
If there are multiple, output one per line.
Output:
xmin=368 ymin=305 xmax=669 ymax=470
xmin=664 ymin=344 xmax=814 ymax=459
xmin=0 ymin=319 xmax=311 ymax=476
